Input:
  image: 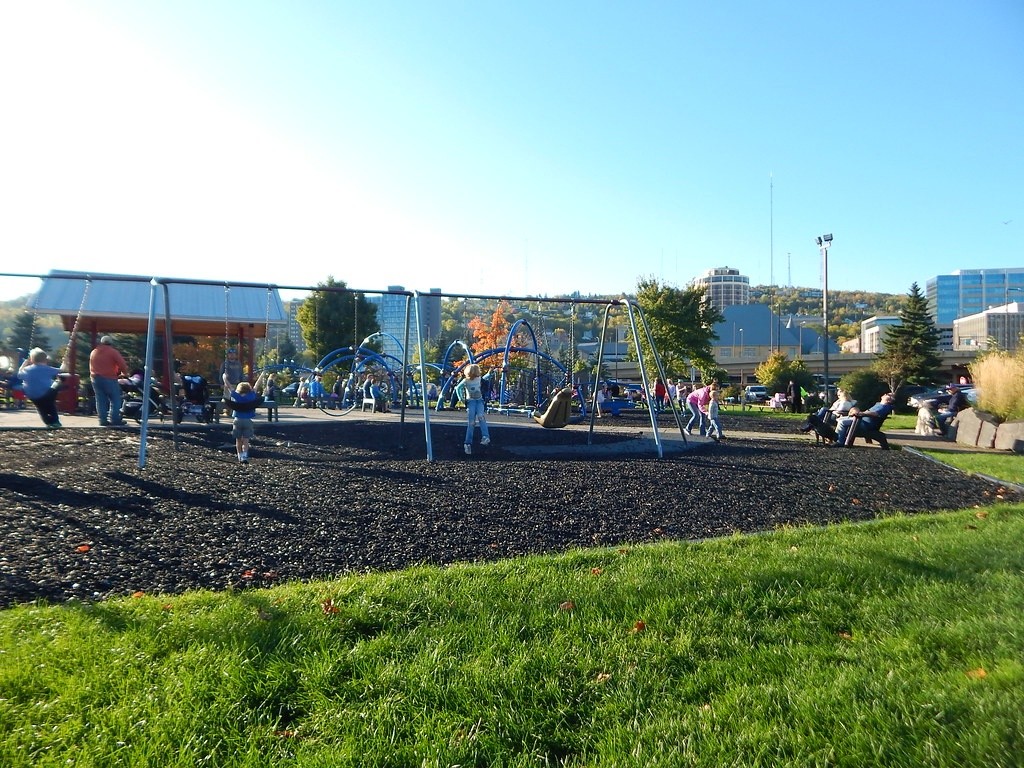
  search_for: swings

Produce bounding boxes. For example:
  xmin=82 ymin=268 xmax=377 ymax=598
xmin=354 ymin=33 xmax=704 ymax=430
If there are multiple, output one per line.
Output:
xmin=27 ymin=278 xmax=91 ymax=405
xmin=532 ymin=301 xmax=574 ymax=428
xmin=222 ymin=286 xmax=270 ymax=411
xmin=461 ymin=297 xmax=501 ymax=407
xmin=315 ymin=291 xmax=357 ymax=417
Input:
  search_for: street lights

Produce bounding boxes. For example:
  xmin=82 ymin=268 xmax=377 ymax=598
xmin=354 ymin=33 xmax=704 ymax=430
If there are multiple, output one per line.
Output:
xmin=816 ymin=233 xmax=833 ymax=405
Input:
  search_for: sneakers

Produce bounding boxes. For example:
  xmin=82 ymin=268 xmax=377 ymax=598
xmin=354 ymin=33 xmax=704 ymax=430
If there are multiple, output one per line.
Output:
xmin=465 ymin=444 xmax=471 ymax=454
xmin=480 ymin=437 xmax=490 ymax=444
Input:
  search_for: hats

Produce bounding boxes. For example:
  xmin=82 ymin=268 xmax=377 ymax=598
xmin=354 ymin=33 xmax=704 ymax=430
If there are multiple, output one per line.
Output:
xmin=101 ymin=335 xmax=112 ymax=344
xmin=945 ymin=383 xmax=957 ymax=390
xmin=678 ymin=380 xmax=683 ymax=382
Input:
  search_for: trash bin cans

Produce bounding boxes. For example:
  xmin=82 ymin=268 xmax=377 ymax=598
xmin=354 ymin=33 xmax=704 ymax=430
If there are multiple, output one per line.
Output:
xmin=55 ymin=374 xmax=81 ymax=414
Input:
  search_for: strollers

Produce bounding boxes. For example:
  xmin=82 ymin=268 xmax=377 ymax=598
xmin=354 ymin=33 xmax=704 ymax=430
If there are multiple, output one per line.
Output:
xmin=117 ymin=370 xmax=165 ymax=425
xmin=174 ymin=374 xmax=217 ymax=426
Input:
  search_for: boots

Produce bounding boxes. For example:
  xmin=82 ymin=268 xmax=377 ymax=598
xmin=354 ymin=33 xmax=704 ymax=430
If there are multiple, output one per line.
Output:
xmin=798 ymin=424 xmax=812 ymax=433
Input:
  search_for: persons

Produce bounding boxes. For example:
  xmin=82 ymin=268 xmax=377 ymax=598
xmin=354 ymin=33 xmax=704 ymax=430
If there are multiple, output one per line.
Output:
xmin=223 ymin=370 xmax=266 ymax=465
xmin=263 ymin=371 xmax=970 ymax=449
xmin=17 ymin=346 xmax=67 ymax=427
xmin=89 ymin=336 xmax=128 ymax=426
xmin=7 ymin=348 xmax=25 ymax=410
xmin=456 ymin=363 xmax=494 ymax=454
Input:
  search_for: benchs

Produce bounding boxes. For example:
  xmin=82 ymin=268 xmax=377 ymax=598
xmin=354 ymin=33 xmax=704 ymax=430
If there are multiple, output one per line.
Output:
xmin=305 ymin=397 xmax=342 ymax=410
xmin=720 ymin=404 xmax=774 ymax=412
xmin=215 ymin=401 xmax=278 ymax=423
xmin=362 ymin=398 xmax=387 ymax=413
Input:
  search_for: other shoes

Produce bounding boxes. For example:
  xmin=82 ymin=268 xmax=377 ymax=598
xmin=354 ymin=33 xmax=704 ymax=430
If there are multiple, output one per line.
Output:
xmin=100 ymin=421 xmax=110 ymax=425
xmin=718 ymin=435 xmax=726 ymax=439
xmin=383 ymin=410 xmax=391 ymax=413
xmin=684 ymin=413 xmax=686 ymax=417
xmin=597 ymin=415 xmax=601 ymax=418
xmin=685 ymin=429 xmax=691 ymax=435
xmin=681 ymin=412 xmax=684 ymax=415
xmin=111 ymin=421 xmax=128 ymax=425
xmin=708 ymin=434 xmax=716 ymax=438
xmin=829 ymin=441 xmax=843 ymax=447
xmin=239 ymin=456 xmax=249 ymax=464
xmin=54 ymin=423 xmax=62 ymax=427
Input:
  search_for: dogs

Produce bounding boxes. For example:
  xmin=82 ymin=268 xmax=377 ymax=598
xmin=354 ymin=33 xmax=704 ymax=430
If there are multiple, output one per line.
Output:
xmin=915 ymin=407 xmax=937 ymax=436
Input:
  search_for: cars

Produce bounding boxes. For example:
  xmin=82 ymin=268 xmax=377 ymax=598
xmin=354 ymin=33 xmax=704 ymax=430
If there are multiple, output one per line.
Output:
xmin=745 ymin=385 xmax=770 ymax=403
xmin=906 ymin=382 xmax=977 ymax=412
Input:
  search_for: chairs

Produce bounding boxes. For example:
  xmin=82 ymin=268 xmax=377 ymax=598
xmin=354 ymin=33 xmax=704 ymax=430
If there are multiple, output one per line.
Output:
xmin=816 ymin=410 xmax=891 ymax=447
